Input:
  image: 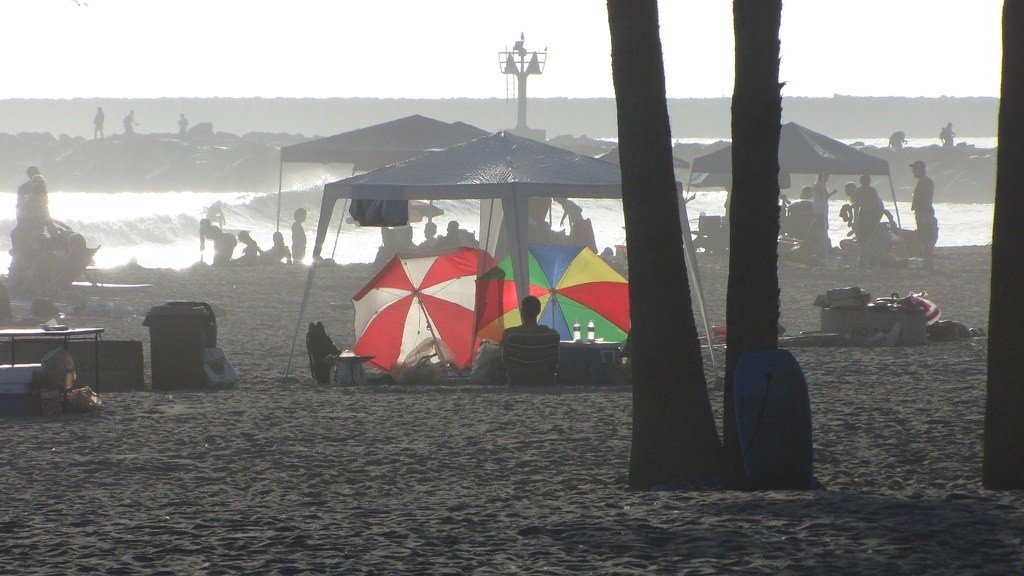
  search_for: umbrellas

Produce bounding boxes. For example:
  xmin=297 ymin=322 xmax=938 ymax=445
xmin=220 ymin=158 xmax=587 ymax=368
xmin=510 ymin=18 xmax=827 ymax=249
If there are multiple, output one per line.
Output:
xmin=349 ymin=246 xmax=505 ymax=372
xmin=473 ymin=244 xmax=631 ymax=369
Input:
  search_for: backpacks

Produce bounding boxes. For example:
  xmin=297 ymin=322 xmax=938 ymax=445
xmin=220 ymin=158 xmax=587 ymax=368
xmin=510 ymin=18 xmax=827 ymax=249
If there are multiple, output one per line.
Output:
xmin=305 ymin=320 xmax=342 ymax=382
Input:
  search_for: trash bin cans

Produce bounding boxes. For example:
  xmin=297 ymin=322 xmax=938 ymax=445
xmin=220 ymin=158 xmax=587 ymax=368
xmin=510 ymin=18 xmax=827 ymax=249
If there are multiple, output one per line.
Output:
xmin=149 ymin=304 xmax=216 ymax=392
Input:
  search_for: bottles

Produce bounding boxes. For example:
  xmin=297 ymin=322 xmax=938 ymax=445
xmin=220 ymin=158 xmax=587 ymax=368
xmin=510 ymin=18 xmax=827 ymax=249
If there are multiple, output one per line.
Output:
xmin=588 ymin=320 xmax=594 ymax=340
xmin=574 ymin=320 xmax=581 ymax=341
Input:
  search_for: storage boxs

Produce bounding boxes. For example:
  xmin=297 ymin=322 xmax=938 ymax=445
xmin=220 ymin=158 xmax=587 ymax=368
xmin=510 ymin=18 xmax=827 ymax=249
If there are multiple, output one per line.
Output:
xmin=558 ymin=339 xmax=620 ymax=380
xmin=0 ymin=363 xmax=42 ymax=415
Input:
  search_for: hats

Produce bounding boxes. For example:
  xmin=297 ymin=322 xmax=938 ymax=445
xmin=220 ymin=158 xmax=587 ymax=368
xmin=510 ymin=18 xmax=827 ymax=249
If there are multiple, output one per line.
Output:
xmin=910 ymin=160 xmax=925 ymax=169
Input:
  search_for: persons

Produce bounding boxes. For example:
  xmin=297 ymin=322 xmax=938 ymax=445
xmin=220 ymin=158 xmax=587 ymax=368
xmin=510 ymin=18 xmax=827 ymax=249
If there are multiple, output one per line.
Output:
xmin=886 ymin=122 xmax=957 ymax=152
xmin=91 ymin=106 xmax=189 ymax=141
xmin=554 ymin=192 xmax=613 ymax=256
xmin=789 ymin=161 xmax=938 ymax=261
xmin=502 ymin=295 xmax=560 ymax=371
xmin=418 ymin=222 xmax=460 ymax=248
xmin=196 ymin=199 xmax=310 ymax=267
xmin=6 ymin=167 xmax=86 ymax=317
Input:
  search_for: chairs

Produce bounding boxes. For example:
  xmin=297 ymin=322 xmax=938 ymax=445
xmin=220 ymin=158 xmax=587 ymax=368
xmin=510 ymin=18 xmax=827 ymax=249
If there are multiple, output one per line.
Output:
xmin=499 ymin=331 xmax=559 ymax=388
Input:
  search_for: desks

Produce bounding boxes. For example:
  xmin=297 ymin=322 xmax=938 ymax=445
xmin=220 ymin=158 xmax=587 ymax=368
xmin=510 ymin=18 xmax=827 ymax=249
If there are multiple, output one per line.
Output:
xmin=0 ymin=328 xmax=104 ymax=395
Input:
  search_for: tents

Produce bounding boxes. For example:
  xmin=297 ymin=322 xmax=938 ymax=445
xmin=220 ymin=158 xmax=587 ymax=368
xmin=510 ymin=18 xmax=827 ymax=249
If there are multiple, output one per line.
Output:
xmin=593 ymin=121 xmax=901 ymax=227
xmin=286 ymin=133 xmax=719 ymax=379
xmin=277 ymin=115 xmax=491 ymax=237
